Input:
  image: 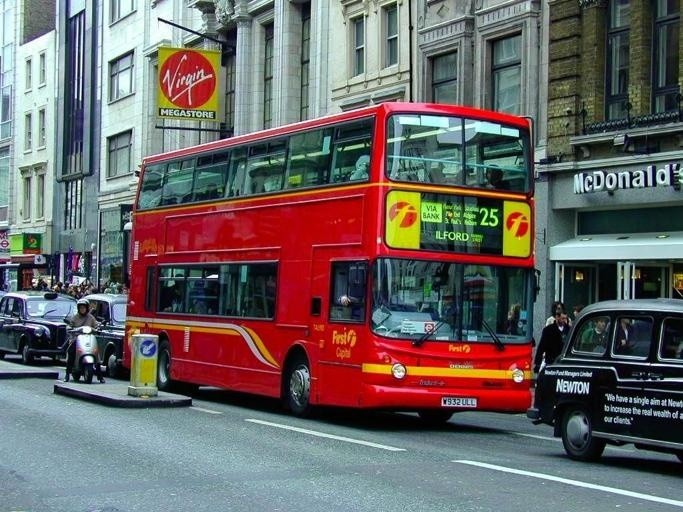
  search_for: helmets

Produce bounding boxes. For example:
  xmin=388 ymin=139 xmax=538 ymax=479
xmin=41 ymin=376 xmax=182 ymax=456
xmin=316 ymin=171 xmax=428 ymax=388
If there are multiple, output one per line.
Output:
xmin=77 ymin=298 xmax=90 ymax=307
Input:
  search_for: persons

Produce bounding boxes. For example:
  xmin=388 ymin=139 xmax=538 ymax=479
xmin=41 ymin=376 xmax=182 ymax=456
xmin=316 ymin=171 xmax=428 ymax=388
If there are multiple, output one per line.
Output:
xmin=531 ymin=302 xmax=634 ymax=425
xmin=337 ymin=295 xmax=351 ymax=307
xmin=508 ymin=302 xmax=526 ymax=335
xmin=28 ymin=279 xmax=130 ymax=302
xmin=159 ymin=274 xmax=184 ymax=312
xmin=63 ymin=298 xmax=106 ymax=383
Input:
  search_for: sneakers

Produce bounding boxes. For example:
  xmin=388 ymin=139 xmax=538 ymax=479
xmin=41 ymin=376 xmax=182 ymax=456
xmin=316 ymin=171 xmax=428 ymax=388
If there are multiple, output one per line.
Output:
xmin=63 ymin=375 xmax=70 ymax=382
xmin=98 ymin=378 xmax=105 ymax=383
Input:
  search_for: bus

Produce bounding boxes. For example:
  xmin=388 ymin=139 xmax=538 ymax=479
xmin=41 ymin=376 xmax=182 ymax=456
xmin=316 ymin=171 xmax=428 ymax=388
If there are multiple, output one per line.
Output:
xmin=123 ymin=102 xmax=535 ymax=422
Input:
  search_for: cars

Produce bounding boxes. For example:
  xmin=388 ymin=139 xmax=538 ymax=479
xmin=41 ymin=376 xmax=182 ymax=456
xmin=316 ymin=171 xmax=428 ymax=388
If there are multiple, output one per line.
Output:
xmin=535 ymin=299 xmax=682 ymax=461
xmin=84 ymin=294 xmax=128 ymax=379
xmin=0 ymin=290 xmax=79 ymax=365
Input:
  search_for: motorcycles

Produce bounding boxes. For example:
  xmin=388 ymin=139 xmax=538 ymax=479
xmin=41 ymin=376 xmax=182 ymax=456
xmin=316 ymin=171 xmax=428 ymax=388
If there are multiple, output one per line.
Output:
xmin=63 ymin=318 xmax=110 ymax=385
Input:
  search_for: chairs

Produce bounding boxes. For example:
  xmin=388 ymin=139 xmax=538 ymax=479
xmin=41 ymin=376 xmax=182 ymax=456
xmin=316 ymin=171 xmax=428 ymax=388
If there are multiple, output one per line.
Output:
xmin=164 ymin=168 xmax=419 ymax=205
